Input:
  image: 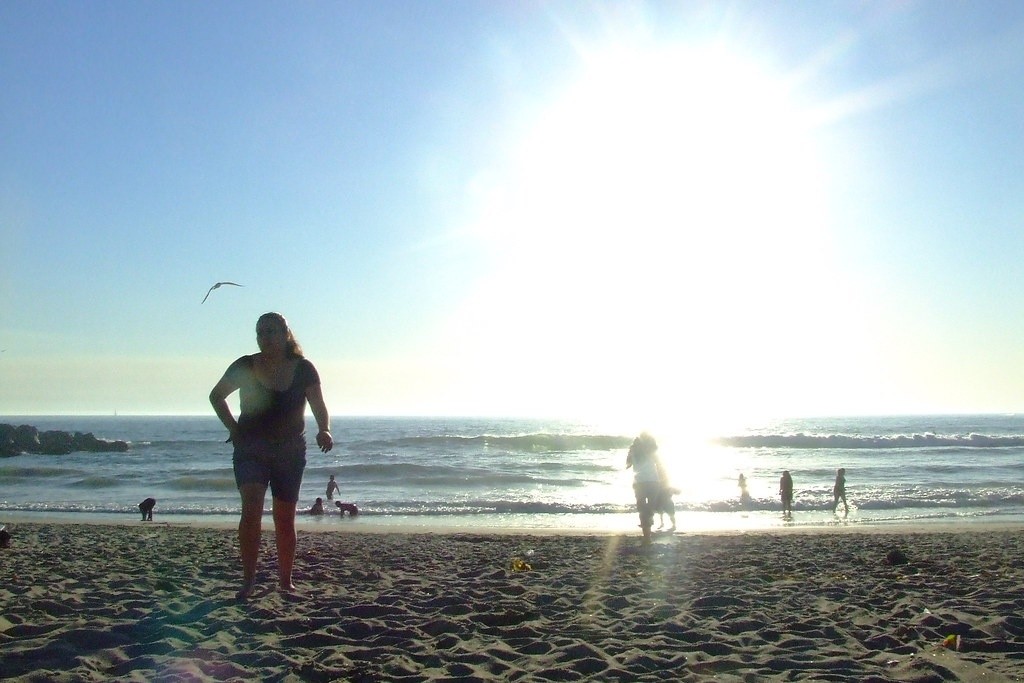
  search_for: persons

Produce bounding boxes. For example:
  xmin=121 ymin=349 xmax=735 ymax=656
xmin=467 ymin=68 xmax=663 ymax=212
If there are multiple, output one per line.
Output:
xmin=625 ymin=430 xmax=680 ymax=545
xmin=139 ymin=498 xmax=155 ymax=521
xmin=209 ymin=312 xmax=334 ymax=600
xmin=310 ymin=474 xmax=358 ymax=516
xmin=738 ymin=467 xmax=850 ymax=519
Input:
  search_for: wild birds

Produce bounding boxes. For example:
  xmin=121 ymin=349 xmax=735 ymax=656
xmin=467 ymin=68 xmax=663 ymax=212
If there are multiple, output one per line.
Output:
xmin=201 ymin=282 xmax=241 ymax=304
